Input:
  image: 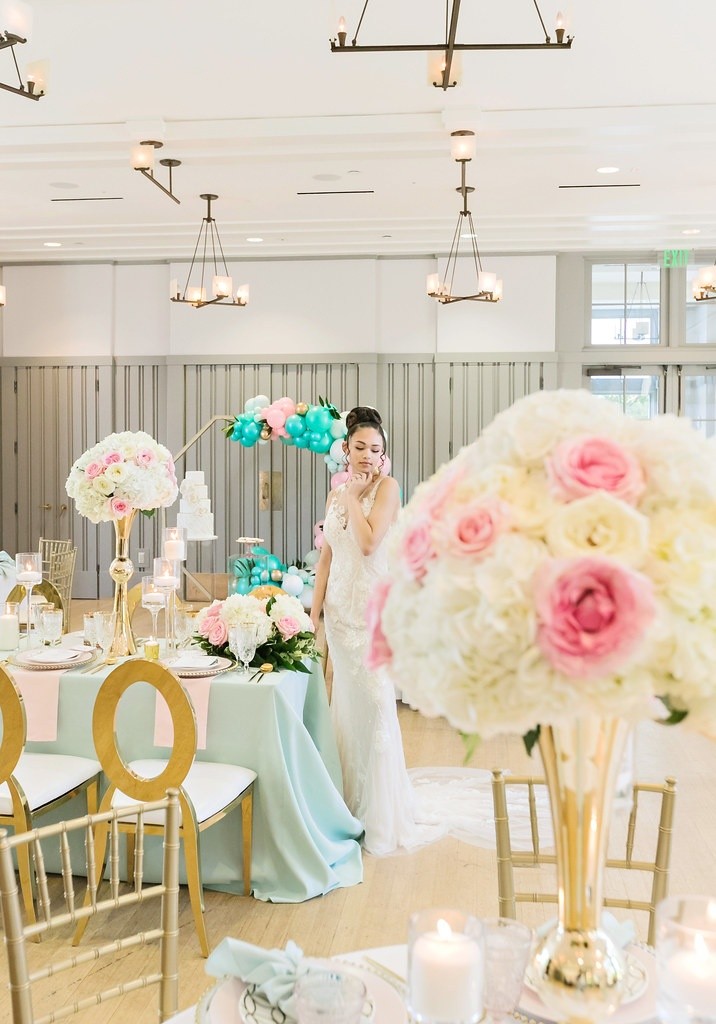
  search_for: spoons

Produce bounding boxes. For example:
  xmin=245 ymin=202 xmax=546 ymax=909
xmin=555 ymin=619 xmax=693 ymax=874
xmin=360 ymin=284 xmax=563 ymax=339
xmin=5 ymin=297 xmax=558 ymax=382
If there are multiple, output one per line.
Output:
xmin=91 ymin=656 xmax=116 ymax=675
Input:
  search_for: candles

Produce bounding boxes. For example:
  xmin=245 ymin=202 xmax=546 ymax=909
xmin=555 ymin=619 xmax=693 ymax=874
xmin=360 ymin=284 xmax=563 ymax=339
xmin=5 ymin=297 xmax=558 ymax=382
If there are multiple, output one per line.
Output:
xmin=20 ymin=559 xmax=40 ymax=582
xmin=163 ymin=532 xmax=183 ymax=555
xmin=144 ymin=585 xmax=166 ymax=604
xmin=160 ymin=563 xmax=174 ymax=585
xmin=426 ymin=920 xmax=474 ymax=978
xmin=0 ymin=604 xmax=16 ymax=650
xmin=674 ymin=921 xmax=714 ymax=1021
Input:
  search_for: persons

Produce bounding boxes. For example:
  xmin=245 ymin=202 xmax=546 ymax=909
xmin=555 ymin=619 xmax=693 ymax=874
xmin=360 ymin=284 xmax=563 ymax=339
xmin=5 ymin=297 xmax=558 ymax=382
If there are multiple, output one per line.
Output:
xmin=310 ymin=407 xmax=410 ymax=855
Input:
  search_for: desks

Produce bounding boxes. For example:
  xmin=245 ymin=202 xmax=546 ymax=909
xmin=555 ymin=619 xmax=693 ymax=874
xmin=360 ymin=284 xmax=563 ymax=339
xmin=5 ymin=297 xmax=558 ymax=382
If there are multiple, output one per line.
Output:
xmin=161 ymin=931 xmax=716 ymax=1023
xmin=11 ymin=637 xmax=369 ymax=902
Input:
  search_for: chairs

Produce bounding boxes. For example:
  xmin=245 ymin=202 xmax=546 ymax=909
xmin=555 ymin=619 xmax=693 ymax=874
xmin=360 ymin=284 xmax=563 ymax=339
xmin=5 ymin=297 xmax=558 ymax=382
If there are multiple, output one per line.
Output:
xmin=1 ymin=789 xmax=191 ymax=1023
xmin=71 ymin=663 xmax=261 ymax=958
xmin=5 ymin=536 xmax=79 ymax=636
xmin=1 ymin=665 xmax=107 ymax=945
xmin=491 ymin=765 xmax=681 ymax=926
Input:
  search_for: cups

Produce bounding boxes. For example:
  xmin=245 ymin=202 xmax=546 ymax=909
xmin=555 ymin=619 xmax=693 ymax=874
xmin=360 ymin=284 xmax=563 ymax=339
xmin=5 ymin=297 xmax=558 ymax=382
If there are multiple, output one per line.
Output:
xmin=143 ymin=643 xmax=160 ymax=659
xmin=0 ymin=601 xmax=20 ymax=651
xmin=405 ymin=907 xmax=487 ymax=1024
xmin=653 ymin=894 xmax=716 ymax=1024
xmin=480 ymin=917 xmax=532 ymax=1024
xmin=296 ymin=972 xmax=367 ymax=1024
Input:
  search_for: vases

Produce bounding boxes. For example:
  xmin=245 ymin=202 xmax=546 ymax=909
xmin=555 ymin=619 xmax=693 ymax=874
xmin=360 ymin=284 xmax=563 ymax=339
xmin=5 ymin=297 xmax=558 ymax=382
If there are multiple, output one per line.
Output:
xmin=516 ymin=705 xmax=643 ymax=1011
xmin=98 ymin=511 xmax=142 ymax=661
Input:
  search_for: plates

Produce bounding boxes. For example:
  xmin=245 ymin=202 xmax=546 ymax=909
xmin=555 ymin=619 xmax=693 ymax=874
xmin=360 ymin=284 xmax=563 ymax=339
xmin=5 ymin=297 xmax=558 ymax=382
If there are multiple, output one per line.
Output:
xmin=7 ymin=647 xmax=97 ymax=670
xmin=168 ymin=655 xmax=238 ymax=679
xmin=194 ymin=958 xmax=409 ymax=1024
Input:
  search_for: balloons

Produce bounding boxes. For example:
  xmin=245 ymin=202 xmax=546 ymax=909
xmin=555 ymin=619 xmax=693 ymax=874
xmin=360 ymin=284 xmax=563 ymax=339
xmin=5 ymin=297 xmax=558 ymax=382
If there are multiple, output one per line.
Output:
xmin=230 ymin=395 xmax=351 ymax=473
xmin=231 ymin=545 xmax=320 ymax=609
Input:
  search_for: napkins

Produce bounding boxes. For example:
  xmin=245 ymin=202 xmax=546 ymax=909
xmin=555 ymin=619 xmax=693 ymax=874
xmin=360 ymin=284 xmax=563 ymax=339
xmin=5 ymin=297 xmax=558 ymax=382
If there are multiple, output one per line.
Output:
xmin=200 ymin=937 xmax=343 ymax=1014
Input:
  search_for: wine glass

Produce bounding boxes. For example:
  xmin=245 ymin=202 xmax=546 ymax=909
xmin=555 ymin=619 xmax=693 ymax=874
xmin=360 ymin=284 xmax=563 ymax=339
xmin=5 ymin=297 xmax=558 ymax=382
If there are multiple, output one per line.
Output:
xmin=83 ymin=611 xmax=98 ymax=648
xmin=141 ymin=575 xmax=167 ymax=642
xmin=15 ymin=552 xmax=42 ymax=650
xmin=153 ymin=557 xmax=181 ymax=659
xmin=174 ymin=603 xmax=194 ymax=651
xmin=161 ymin=527 xmax=187 ymax=659
xmin=228 ymin=622 xmax=261 ymax=677
xmin=42 ymin=608 xmax=63 ymax=647
xmin=93 ymin=610 xmax=117 ymax=662
xmin=31 ymin=601 xmax=55 ymax=647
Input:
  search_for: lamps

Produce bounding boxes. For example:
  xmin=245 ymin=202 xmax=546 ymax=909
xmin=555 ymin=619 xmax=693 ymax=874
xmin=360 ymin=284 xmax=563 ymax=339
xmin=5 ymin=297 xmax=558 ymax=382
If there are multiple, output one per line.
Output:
xmin=427 ymin=131 xmax=506 ymax=303
xmin=134 ymin=140 xmax=182 ymax=205
xmin=329 ymin=0 xmax=576 ymax=92
xmin=0 ymin=30 xmax=47 ymax=101
xmin=692 ymin=263 xmax=716 ymax=303
xmin=171 ymin=193 xmax=250 ymax=309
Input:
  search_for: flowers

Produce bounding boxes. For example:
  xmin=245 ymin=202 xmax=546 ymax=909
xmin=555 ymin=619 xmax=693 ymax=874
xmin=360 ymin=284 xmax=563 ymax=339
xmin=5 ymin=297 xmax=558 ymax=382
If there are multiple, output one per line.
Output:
xmin=193 ymin=589 xmax=318 ymax=672
xmin=65 ymin=427 xmax=178 ymax=528
xmin=363 ymin=393 xmax=715 ymax=740
